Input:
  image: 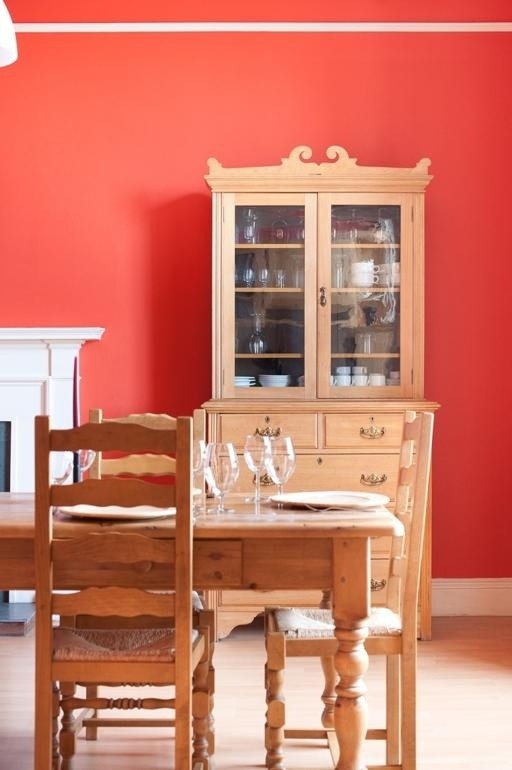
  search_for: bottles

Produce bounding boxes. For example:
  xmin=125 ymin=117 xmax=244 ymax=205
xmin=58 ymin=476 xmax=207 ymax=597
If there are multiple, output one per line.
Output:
xmin=246 ymin=313 xmax=269 ymax=354
xmin=238 ymin=207 xmax=368 ymax=246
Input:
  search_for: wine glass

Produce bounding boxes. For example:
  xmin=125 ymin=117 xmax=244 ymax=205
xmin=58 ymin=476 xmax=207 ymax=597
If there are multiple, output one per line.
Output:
xmin=49 ymin=450 xmax=97 ymax=520
xmin=190 ymin=434 xmax=297 ymax=521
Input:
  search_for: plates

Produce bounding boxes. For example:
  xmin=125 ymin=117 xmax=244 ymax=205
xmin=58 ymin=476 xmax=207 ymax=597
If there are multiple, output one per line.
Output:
xmin=234 ymin=374 xmax=292 ymax=387
xmin=55 ymin=504 xmax=176 ymax=522
xmin=271 ymin=491 xmax=392 ymax=510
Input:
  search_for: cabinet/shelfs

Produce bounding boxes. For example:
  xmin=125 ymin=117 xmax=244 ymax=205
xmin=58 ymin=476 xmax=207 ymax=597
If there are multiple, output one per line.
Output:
xmin=200 ymin=144 xmax=441 ymax=644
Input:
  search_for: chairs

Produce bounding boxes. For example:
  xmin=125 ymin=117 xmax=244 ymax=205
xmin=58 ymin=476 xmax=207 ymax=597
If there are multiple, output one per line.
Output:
xmin=32 ymin=415 xmax=216 ymax=769
xmin=85 ymin=408 xmax=215 ymax=757
xmin=265 ymin=408 xmax=436 ymax=770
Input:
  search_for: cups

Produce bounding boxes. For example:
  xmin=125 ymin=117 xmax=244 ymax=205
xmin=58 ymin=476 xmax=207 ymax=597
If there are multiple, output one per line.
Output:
xmin=328 ymin=366 xmax=387 ymax=389
xmin=242 ymin=268 xmax=292 ymax=290
xmin=347 ymin=262 xmax=399 ymax=288
